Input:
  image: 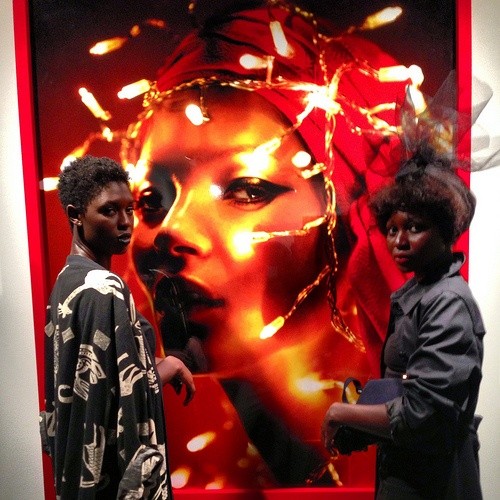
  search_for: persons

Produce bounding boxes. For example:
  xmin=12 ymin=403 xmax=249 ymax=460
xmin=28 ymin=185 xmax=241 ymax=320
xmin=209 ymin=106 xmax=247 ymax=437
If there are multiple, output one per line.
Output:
xmin=322 ymin=73 xmax=500 ymax=500
xmin=123 ymin=7 xmax=439 ymax=489
xmin=39 ymin=155 xmax=196 ymax=500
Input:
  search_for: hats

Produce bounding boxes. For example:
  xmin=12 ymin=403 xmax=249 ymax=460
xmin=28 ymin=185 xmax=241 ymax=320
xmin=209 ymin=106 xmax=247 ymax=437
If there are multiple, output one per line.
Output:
xmin=145 ymin=0 xmax=420 ymax=371
